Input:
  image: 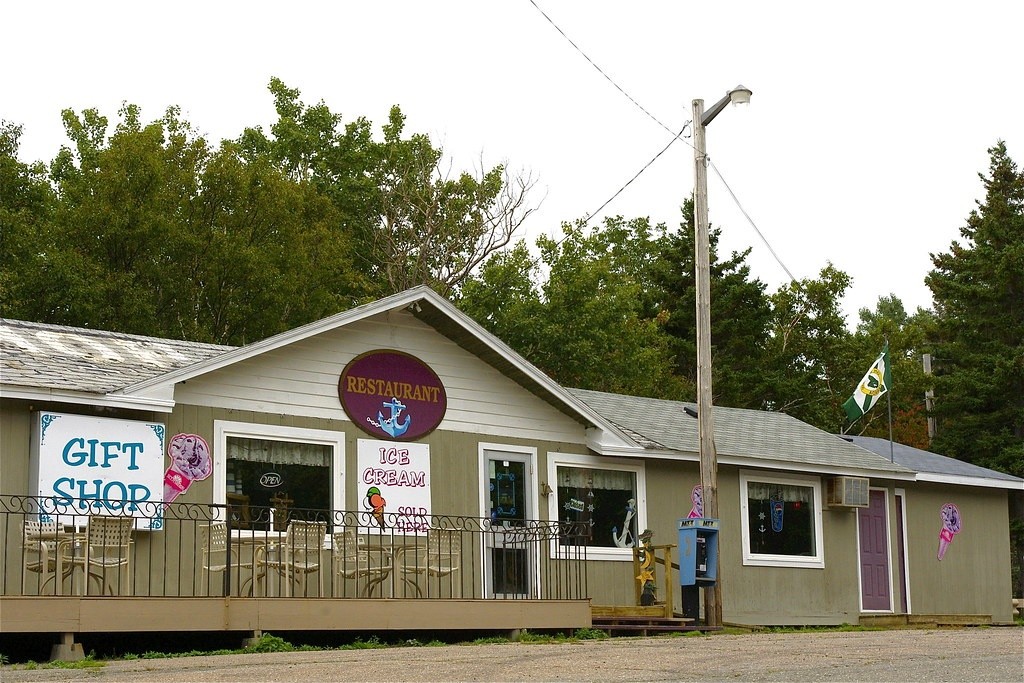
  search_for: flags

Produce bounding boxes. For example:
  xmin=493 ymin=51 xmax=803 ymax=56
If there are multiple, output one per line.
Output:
xmin=841 ymin=345 xmax=892 ymax=424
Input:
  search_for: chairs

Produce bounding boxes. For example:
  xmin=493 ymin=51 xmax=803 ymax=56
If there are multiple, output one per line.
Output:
xmin=226 ymin=492 xmax=253 ymax=530
xmin=19 ymin=514 xmax=464 ymax=599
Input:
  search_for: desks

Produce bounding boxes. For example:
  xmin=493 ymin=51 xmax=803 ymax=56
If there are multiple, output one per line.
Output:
xmin=27 ymin=532 xmax=134 ymax=595
xmin=358 ymin=543 xmax=431 ymax=598
xmin=230 ymin=536 xmax=324 ymax=597
xmin=270 ymin=498 xmax=294 ymax=531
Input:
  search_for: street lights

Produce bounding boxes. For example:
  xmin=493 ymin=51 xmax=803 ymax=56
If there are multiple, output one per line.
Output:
xmin=693 ymin=84 xmax=753 ymax=628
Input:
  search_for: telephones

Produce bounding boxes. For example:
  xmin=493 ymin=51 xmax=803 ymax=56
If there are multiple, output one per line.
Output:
xmin=696 ymin=543 xmax=708 ymax=574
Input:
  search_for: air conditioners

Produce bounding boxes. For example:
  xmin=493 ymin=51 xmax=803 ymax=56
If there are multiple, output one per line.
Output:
xmin=826 ymin=476 xmax=870 ymax=508
